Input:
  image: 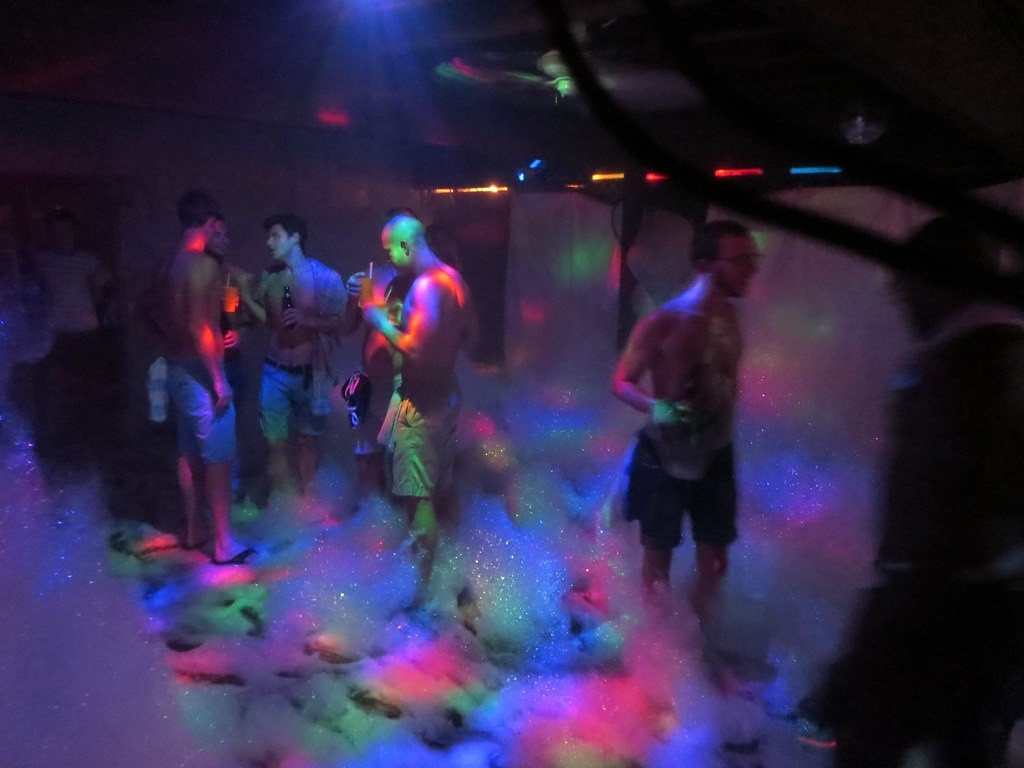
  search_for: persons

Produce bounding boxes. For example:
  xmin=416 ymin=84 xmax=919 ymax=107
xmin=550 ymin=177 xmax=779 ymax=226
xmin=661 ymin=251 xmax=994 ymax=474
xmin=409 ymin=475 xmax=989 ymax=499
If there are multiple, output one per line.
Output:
xmin=235 ymin=214 xmax=347 ymax=496
xmin=796 ymin=202 xmax=1024 ymax=768
xmin=207 ymin=215 xmax=249 ymax=505
xmin=612 ymin=219 xmax=758 ymax=600
xmin=346 ymin=207 xmax=480 ymax=612
xmin=24 ymin=220 xmax=113 ymax=419
xmin=150 ymin=189 xmax=258 ymax=565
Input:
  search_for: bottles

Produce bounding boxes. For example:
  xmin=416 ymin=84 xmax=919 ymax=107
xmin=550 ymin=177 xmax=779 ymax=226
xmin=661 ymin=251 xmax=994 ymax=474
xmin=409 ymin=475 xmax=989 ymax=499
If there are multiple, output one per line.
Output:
xmin=283 ymin=285 xmax=295 ymax=329
xmin=683 ymin=365 xmax=702 ymax=401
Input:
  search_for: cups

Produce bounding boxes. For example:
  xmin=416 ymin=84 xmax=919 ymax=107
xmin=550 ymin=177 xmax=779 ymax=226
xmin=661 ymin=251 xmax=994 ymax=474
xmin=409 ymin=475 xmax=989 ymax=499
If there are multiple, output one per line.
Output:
xmin=359 ymin=278 xmax=376 ymax=298
xmin=222 ymin=287 xmax=237 ymax=312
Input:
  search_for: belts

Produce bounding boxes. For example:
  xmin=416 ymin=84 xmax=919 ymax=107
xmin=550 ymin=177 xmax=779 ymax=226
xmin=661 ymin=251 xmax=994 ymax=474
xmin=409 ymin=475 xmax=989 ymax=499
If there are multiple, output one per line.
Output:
xmin=262 ymin=356 xmax=314 ymax=376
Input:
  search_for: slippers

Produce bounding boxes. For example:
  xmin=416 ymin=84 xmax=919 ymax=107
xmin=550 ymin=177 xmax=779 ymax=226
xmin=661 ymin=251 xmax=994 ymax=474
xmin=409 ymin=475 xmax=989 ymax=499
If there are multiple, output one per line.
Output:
xmin=181 ymin=536 xmax=213 ymax=550
xmin=211 ymin=547 xmax=261 ymax=565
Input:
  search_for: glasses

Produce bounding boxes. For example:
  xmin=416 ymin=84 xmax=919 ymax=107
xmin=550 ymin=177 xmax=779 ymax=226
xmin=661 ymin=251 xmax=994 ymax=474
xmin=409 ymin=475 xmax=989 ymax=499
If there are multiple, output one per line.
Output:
xmin=717 ymin=251 xmax=763 ymax=266
